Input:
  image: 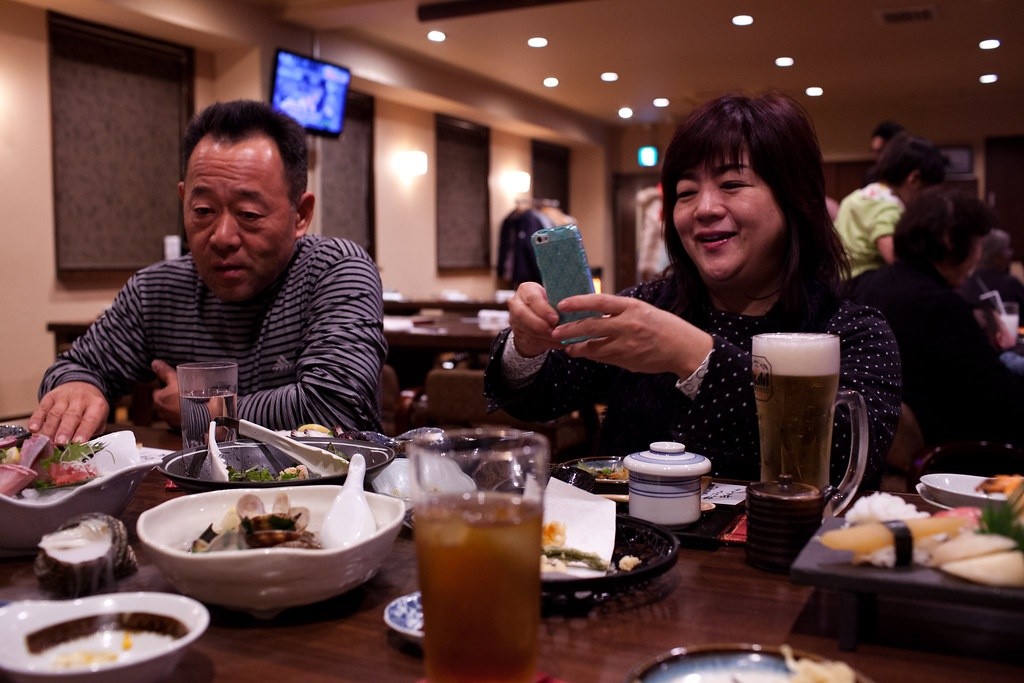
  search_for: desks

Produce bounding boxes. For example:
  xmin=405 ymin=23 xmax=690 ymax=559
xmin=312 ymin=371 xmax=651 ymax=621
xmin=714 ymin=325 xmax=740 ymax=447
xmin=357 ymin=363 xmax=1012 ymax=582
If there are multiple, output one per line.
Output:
xmin=45 ymin=306 xmax=511 ymax=365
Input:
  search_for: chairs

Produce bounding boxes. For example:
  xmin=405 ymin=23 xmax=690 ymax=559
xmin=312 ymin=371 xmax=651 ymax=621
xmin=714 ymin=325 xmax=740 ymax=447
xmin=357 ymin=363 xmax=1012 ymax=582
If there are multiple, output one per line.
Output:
xmin=427 ymin=369 xmax=554 ymax=451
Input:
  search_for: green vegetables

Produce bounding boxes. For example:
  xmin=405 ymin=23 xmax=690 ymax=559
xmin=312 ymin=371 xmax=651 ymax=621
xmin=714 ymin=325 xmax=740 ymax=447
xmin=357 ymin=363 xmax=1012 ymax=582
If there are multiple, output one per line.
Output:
xmin=225 ymin=463 xmax=301 ymax=482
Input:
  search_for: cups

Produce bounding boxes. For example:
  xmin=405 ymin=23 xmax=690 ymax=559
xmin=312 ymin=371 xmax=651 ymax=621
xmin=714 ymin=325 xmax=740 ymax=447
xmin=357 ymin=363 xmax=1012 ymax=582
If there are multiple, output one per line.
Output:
xmin=623 ymin=442 xmax=711 ymax=529
xmin=177 ymin=360 xmax=239 ymax=449
xmin=496 ymin=291 xmax=515 ymax=303
xmin=478 ymin=309 xmax=510 ymax=331
xmin=752 ymin=332 xmax=869 ymax=525
xmin=403 ymin=427 xmax=549 ymax=683
xmin=996 ymin=302 xmax=1019 ymax=349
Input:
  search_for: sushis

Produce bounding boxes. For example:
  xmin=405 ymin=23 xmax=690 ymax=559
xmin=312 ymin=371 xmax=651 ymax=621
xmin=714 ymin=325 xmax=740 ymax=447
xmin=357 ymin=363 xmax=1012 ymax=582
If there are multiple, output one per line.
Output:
xmin=817 ymin=492 xmax=1023 ymax=589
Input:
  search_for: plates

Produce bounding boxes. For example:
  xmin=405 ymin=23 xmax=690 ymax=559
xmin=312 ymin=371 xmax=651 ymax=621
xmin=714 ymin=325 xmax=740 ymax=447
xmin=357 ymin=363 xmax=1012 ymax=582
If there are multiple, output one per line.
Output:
xmin=915 ymin=484 xmax=954 ymax=511
xmin=548 ymin=460 xmax=597 ymax=493
xmin=625 ymin=643 xmax=875 ymax=683
xmin=701 ymin=500 xmax=716 ymax=512
xmin=385 ymin=589 xmax=424 ymax=647
xmin=559 ymin=455 xmax=629 ymax=494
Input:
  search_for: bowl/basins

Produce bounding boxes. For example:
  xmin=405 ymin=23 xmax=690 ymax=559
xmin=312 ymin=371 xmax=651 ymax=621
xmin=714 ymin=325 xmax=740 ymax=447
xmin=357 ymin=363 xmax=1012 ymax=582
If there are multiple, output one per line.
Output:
xmin=0 ymin=591 xmax=212 ymax=683
xmin=158 ymin=436 xmax=396 ymax=495
xmin=0 ymin=430 xmax=163 ymax=558
xmin=920 ymin=472 xmax=1008 ymax=510
xmin=135 ymin=485 xmax=406 ymax=611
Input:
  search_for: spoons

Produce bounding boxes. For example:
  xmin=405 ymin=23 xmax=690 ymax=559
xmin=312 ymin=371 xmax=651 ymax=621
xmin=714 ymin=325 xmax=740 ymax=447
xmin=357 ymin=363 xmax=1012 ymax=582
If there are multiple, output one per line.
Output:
xmin=318 ymin=453 xmax=377 ymax=549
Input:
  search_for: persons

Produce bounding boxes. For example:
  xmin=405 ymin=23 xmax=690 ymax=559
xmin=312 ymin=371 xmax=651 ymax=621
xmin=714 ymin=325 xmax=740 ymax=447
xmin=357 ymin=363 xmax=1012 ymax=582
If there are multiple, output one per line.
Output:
xmin=870 ymin=120 xmax=921 ymax=166
xmin=280 ymin=70 xmax=320 ymax=124
xmin=844 ymin=183 xmax=1024 ymax=477
xmin=634 ymin=185 xmax=664 ymax=281
xmin=314 ymin=78 xmax=333 ymax=123
xmin=971 ymin=228 xmax=1024 ymax=329
xmin=28 ymin=96 xmax=389 ymax=446
xmin=834 ymin=130 xmax=949 ymax=279
xmin=483 ymin=86 xmax=901 ymax=491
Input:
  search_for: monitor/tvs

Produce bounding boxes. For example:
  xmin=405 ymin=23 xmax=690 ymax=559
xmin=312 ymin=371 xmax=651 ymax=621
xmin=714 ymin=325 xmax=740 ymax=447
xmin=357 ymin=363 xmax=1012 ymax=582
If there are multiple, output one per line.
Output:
xmin=269 ymin=49 xmax=351 ymax=138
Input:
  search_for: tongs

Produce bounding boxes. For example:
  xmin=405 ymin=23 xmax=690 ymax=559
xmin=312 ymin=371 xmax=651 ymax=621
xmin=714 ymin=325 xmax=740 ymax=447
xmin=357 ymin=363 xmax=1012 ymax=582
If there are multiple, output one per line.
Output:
xmin=209 ymin=416 xmax=350 ymax=482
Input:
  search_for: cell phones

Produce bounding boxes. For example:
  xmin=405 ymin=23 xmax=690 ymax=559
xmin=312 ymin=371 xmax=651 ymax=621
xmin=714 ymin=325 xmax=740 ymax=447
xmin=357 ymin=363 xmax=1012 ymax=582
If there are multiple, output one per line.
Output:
xmin=530 ymin=225 xmax=604 ymax=345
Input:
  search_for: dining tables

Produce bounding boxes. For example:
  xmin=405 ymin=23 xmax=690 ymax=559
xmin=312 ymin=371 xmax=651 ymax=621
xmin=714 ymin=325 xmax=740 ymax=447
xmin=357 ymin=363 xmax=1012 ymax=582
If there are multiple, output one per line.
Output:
xmin=0 ymin=475 xmax=1024 ymax=683
xmin=383 ymin=300 xmax=512 ymax=314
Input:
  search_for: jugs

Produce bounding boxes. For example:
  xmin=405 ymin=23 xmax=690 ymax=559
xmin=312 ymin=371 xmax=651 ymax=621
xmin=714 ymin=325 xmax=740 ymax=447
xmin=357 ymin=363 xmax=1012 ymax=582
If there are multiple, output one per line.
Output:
xmin=745 ymin=474 xmax=840 ymax=573
